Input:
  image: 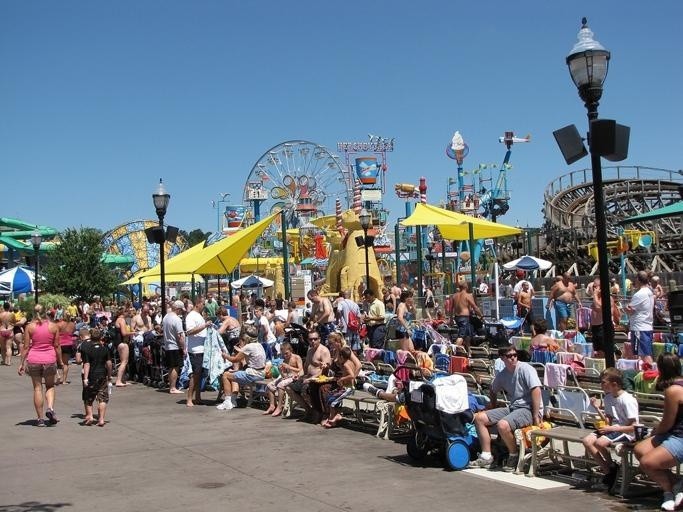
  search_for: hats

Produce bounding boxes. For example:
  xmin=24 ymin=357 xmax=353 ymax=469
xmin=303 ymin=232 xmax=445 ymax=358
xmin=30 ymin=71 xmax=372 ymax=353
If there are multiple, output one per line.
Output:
xmin=171 ymin=299 xmax=186 ymax=312
xmin=240 ymin=325 xmax=258 ymax=342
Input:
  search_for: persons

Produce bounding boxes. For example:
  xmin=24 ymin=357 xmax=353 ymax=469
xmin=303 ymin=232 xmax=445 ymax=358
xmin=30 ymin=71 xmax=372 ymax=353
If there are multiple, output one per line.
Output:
xmin=201 ymin=286 xmax=432 ymax=436
xmin=1 ymin=294 xmax=215 ymax=408
xmin=17 ymin=304 xmax=65 ymax=427
xmin=74 ymin=326 xmax=112 ymax=426
xmin=443 ymin=270 xmax=683 ymax=511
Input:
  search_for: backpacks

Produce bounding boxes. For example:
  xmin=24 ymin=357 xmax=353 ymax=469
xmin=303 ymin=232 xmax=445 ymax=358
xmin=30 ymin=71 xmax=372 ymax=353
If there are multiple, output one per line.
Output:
xmin=345 ymin=310 xmax=359 ymax=330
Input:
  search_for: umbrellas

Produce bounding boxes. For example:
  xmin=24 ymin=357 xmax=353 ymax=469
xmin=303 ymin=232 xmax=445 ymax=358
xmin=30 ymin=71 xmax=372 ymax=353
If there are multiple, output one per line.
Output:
xmin=502 ymin=254 xmax=552 ymax=279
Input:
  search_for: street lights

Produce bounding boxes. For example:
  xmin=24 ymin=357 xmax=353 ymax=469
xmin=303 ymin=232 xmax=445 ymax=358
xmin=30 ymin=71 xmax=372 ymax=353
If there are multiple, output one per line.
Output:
xmin=357 ymin=206 xmax=372 ymax=291
xmin=564 ymin=15 xmax=613 ymax=372
xmin=149 ymin=176 xmax=171 ymax=323
xmin=424 ymin=235 xmax=435 ymax=292
xmin=29 ymin=223 xmax=46 ymax=307
xmin=514 ymin=219 xmax=521 ymax=259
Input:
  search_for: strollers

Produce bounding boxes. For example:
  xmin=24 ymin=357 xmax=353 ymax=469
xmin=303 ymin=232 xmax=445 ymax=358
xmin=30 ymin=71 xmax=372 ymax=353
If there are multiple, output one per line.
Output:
xmin=102 ymin=326 xmax=188 ymax=388
xmin=391 ymin=365 xmax=502 ymax=472
xmin=282 ymin=324 xmax=311 ymax=355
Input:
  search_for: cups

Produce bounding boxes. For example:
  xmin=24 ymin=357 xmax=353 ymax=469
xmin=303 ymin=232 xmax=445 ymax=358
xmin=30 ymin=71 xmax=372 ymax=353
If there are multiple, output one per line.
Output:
xmin=633 ymin=423 xmax=644 ymax=441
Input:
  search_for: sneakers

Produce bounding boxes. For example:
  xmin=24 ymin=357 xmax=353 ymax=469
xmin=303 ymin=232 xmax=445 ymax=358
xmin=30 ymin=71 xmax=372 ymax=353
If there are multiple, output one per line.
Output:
xmin=500 ymin=454 xmax=518 ymax=472
xmin=464 ymin=451 xmax=496 ymax=470
xmin=95 ymin=419 xmax=105 ymax=426
xmin=43 ymin=408 xmax=58 ymax=425
xmin=32 ymin=418 xmax=45 ymax=427
xmin=214 ymin=394 xmax=238 ymax=410
xmin=660 ymin=490 xmax=674 ymax=511
xmin=82 ymin=417 xmax=93 ymax=425
xmin=671 ymin=480 xmax=683 ymax=512
xmin=360 ymin=381 xmax=383 ymax=398
xmin=602 ymin=461 xmax=621 ymax=495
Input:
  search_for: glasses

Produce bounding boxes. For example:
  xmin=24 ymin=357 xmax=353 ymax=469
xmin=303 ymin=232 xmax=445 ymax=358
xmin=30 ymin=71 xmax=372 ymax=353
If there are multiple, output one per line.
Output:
xmin=309 ymin=337 xmax=320 ymax=341
xmin=503 ymin=352 xmax=518 ymax=359
